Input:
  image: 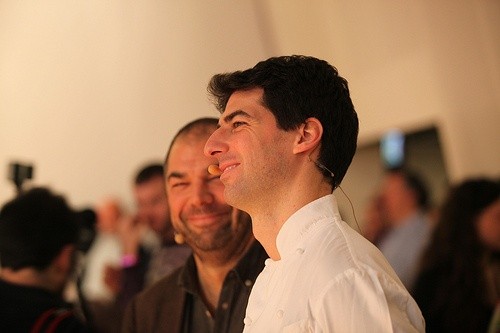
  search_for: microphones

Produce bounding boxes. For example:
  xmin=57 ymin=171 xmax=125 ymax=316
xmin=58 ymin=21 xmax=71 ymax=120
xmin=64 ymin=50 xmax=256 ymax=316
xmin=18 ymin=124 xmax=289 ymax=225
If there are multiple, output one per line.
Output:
xmin=174 ymin=232 xmax=184 ymax=244
xmin=208 ymin=164 xmax=222 ymax=176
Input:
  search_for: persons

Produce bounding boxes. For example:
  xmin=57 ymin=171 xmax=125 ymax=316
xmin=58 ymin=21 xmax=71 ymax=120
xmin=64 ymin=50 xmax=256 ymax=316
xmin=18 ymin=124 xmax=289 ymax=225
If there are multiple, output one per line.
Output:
xmin=0 ymin=160 xmax=191 ymax=332
xmin=204 ymin=53 xmax=425 ymax=333
xmin=365 ymin=164 xmax=500 ymax=333
xmin=120 ymin=117 xmax=270 ymax=333
